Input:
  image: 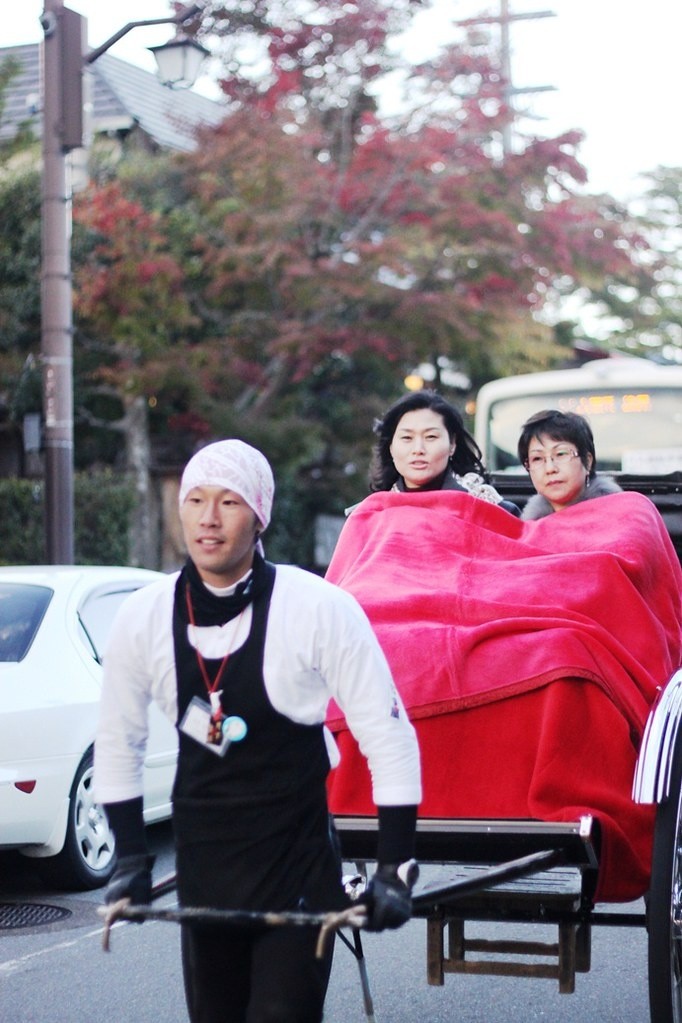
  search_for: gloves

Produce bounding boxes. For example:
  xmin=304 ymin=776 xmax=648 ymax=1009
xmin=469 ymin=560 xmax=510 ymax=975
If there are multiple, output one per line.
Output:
xmin=106 ymin=854 xmax=157 ymax=926
xmin=358 ymin=850 xmax=419 ymax=934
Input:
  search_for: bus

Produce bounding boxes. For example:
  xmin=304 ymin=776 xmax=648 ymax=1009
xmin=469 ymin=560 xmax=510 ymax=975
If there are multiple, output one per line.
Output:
xmin=473 ymin=356 xmax=682 ymax=476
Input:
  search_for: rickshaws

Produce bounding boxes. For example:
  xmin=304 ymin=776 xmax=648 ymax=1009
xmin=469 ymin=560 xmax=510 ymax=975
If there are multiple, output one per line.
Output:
xmin=95 ymin=473 xmax=682 ymax=1022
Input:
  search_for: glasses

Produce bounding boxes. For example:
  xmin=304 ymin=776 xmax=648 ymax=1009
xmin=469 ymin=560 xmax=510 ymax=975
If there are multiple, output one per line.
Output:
xmin=518 ymin=447 xmax=581 ymax=469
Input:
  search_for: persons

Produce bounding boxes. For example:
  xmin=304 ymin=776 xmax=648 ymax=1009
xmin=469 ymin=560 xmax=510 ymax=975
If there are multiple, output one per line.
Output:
xmin=92 ymin=433 xmax=424 ymax=1023
xmin=511 ymin=412 xmax=625 ymax=530
xmin=334 ymin=387 xmax=503 ymax=513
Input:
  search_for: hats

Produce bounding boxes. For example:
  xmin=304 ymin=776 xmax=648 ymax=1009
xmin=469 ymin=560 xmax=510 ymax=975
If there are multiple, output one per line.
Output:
xmin=182 ymin=440 xmax=275 ymax=536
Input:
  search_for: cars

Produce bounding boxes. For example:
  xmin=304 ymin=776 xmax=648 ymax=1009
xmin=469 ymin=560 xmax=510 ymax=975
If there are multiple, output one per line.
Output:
xmin=0 ymin=564 xmax=171 ymax=890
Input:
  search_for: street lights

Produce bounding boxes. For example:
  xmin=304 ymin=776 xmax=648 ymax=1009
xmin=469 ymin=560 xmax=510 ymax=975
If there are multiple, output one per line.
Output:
xmin=40 ymin=0 xmax=213 ymax=559
